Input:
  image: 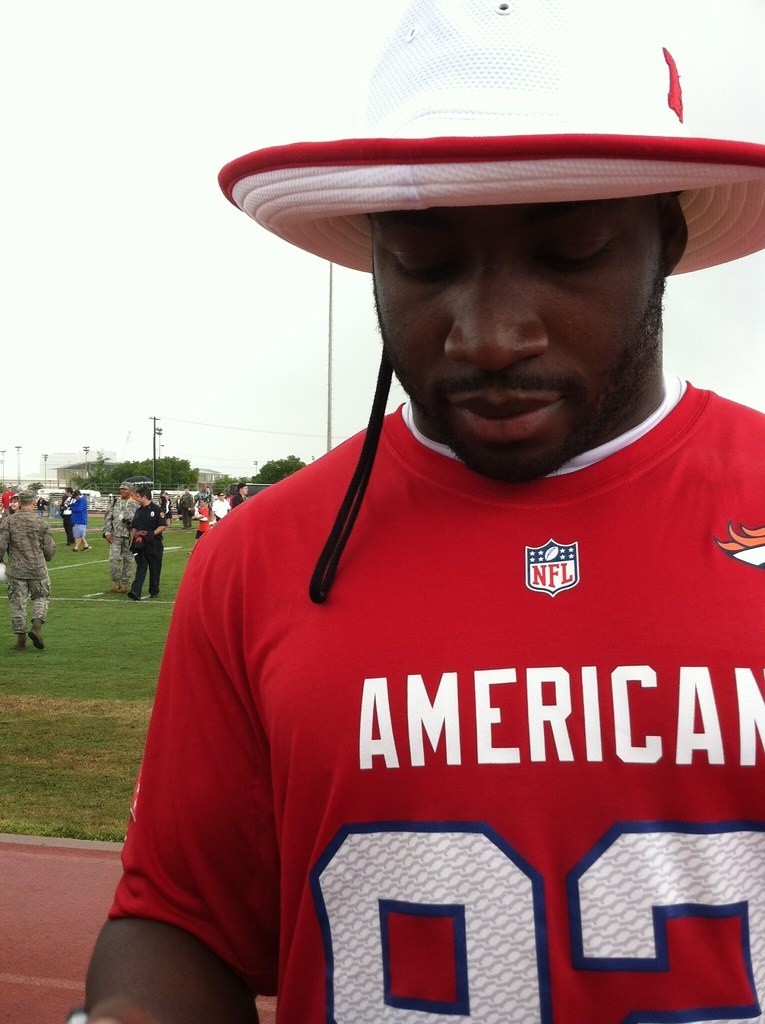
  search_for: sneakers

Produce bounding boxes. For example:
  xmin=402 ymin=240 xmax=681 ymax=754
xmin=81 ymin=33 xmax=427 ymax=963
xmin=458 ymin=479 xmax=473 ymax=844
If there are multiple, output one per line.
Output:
xmin=16 ymin=633 xmax=26 ymax=649
xmin=28 ymin=620 xmax=46 ymax=649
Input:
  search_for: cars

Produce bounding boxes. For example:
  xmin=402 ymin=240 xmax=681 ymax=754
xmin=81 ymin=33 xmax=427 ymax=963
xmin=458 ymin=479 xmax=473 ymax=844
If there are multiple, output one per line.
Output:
xmin=33 ymin=488 xmax=100 ymax=505
xmin=224 ymin=483 xmax=273 ymax=502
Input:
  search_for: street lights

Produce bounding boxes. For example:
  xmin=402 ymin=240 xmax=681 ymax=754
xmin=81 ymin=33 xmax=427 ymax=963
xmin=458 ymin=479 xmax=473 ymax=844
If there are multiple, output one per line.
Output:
xmin=0 ymin=450 xmax=7 ymax=484
xmin=149 ymin=416 xmax=162 ymax=490
xmin=154 ymin=427 xmax=163 ymax=459
xmin=42 ymin=453 xmax=48 ymax=487
xmin=83 ymin=447 xmax=90 ymax=470
xmin=15 ymin=446 xmax=22 ymax=485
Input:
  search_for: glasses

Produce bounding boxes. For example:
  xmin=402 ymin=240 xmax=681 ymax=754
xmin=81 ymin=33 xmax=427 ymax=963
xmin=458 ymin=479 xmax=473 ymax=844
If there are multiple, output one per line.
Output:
xmin=219 ymin=495 xmax=224 ymax=496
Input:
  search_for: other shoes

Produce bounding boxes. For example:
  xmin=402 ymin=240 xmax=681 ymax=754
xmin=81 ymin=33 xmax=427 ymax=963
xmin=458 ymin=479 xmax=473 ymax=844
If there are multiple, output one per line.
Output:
xmin=82 ymin=545 xmax=92 ymax=552
xmin=70 ymin=548 xmax=79 ymax=553
xmin=183 ymin=528 xmax=187 ymax=529
xmin=121 ymin=583 xmax=129 ymax=593
xmin=110 ymin=582 xmax=121 ymax=592
xmin=149 ymin=590 xmax=159 ymax=598
xmin=188 ymin=525 xmax=192 ymax=527
xmin=127 ymin=590 xmax=141 ymax=601
xmin=67 ymin=542 xmax=71 ymax=545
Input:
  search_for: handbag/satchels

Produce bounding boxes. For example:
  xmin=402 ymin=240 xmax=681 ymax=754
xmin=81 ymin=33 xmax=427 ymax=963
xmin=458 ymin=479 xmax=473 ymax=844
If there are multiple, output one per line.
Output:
xmin=103 ymin=532 xmax=106 ymax=539
xmin=63 ymin=508 xmax=72 ymax=515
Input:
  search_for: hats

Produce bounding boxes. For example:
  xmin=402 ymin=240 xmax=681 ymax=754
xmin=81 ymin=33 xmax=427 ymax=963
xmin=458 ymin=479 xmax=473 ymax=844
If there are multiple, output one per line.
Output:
xmin=119 ymin=482 xmax=131 ymax=488
xmin=236 ymin=483 xmax=246 ymax=492
xmin=218 ymin=1 xmax=765 ymax=277
xmin=18 ymin=490 xmax=35 ymax=501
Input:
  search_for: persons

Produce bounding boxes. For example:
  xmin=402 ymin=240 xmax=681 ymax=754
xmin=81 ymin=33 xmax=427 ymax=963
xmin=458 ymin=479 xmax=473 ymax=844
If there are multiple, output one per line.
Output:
xmin=59 ymin=487 xmax=92 ymax=552
xmin=0 ymin=486 xmax=57 ymax=649
xmin=103 ymin=481 xmax=249 ymax=601
xmin=81 ymin=0 xmax=765 ymax=1024
